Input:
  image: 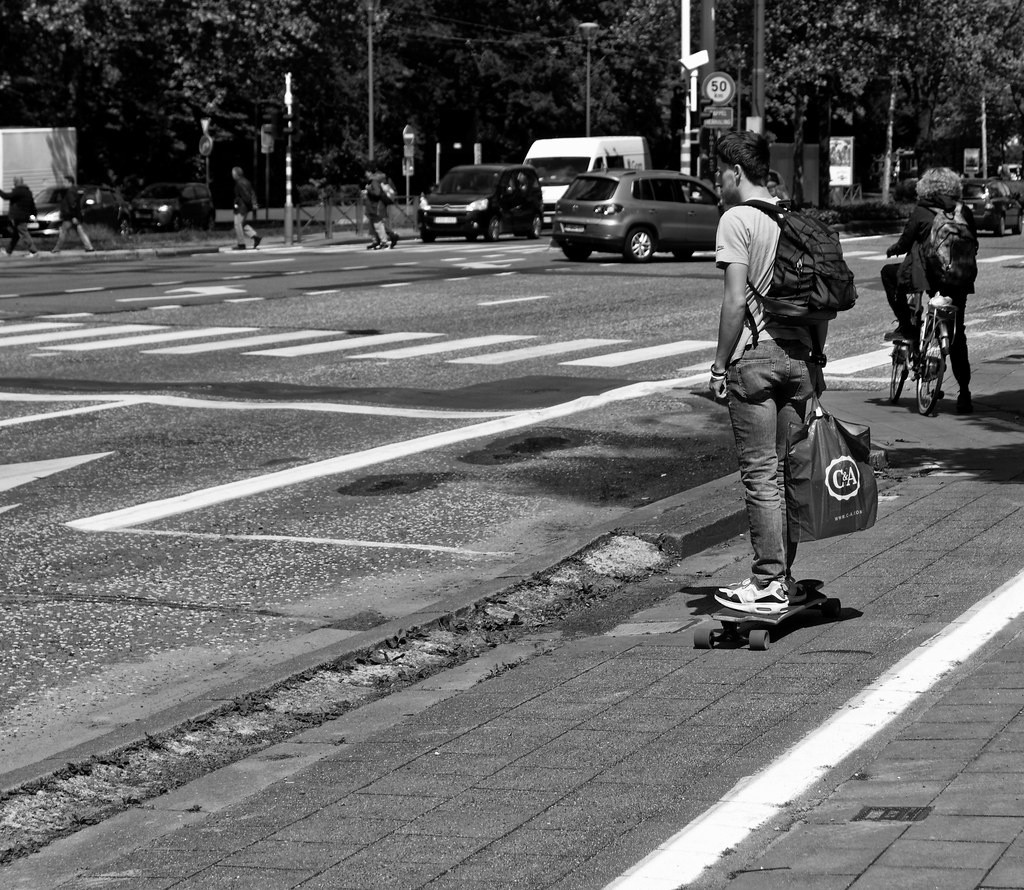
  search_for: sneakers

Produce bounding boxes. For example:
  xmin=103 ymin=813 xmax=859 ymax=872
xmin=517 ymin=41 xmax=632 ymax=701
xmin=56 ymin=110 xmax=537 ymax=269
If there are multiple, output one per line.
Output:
xmin=785 ymin=577 xmax=807 ymax=602
xmin=713 ymin=575 xmax=789 ymax=614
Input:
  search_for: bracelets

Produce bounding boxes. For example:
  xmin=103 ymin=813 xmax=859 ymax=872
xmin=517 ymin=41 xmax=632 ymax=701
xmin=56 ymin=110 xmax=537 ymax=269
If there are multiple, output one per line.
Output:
xmin=711 ymin=364 xmax=727 ymax=380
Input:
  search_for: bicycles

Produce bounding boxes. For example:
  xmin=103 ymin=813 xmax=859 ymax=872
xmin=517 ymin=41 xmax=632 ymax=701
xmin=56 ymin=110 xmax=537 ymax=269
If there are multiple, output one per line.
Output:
xmin=889 ymin=290 xmax=958 ymax=416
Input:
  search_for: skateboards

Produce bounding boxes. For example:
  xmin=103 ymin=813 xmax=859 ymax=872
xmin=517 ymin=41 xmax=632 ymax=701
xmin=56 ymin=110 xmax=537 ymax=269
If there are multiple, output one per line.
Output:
xmin=692 ymin=579 xmax=842 ymax=650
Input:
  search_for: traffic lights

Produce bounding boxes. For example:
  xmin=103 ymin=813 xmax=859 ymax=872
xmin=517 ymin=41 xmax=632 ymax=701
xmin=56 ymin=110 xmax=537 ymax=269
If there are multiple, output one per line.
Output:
xmin=689 ymin=95 xmax=713 ymax=130
xmin=262 ymin=108 xmax=298 ymax=142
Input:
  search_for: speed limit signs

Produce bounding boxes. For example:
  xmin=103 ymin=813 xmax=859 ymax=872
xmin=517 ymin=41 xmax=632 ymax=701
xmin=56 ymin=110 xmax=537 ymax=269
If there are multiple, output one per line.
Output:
xmin=703 ymin=71 xmax=736 ymax=107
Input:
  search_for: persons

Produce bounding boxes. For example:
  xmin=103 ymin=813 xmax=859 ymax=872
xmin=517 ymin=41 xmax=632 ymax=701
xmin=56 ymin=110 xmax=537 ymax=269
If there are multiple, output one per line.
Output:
xmin=362 ymin=165 xmax=398 ymax=249
xmin=52 ymin=176 xmax=94 ymax=252
xmin=0 ymin=176 xmax=37 ymax=258
xmin=710 ymin=134 xmax=830 ymax=614
xmin=232 ymin=168 xmax=262 ymax=250
xmin=881 ymin=167 xmax=978 ymax=402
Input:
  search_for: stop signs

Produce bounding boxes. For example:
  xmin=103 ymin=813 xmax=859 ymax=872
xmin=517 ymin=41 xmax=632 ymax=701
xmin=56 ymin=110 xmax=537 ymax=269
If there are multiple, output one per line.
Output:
xmin=402 ymin=125 xmax=415 ymax=145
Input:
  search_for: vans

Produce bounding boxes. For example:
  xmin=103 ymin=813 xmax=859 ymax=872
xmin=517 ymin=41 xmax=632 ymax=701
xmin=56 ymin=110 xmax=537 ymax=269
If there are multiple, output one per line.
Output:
xmin=516 ymin=135 xmax=653 ymax=225
xmin=417 ymin=164 xmax=543 ymax=243
xmin=130 ymin=181 xmax=216 ymax=231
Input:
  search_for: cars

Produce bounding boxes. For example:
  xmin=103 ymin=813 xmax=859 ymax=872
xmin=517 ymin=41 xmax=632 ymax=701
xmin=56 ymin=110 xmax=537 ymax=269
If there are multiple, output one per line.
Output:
xmin=551 ymin=168 xmax=725 ymax=264
xmin=6 ymin=185 xmax=132 ymax=239
xmin=960 ymin=177 xmax=1024 ymax=237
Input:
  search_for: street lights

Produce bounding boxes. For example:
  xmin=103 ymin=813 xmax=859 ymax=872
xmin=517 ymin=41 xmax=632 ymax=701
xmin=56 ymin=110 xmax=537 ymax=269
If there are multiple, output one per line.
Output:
xmin=577 ymin=22 xmax=600 ymax=138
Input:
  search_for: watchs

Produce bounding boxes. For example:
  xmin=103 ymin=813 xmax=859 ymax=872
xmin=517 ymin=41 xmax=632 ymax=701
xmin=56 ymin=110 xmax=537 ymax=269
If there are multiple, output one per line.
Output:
xmin=814 ymin=354 xmax=827 ymax=367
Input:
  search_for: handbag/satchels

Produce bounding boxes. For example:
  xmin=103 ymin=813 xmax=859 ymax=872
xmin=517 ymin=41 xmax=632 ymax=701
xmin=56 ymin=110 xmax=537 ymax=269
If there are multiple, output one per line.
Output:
xmin=785 ymin=392 xmax=879 ymax=542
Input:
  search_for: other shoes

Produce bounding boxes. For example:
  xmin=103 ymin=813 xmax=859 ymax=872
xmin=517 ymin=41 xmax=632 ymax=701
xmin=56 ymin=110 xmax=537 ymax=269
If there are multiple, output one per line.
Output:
xmin=85 ymin=249 xmax=94 ymax=253
xmin=367 ymin=243 xmax=380 ymax=249
xmin=0 ymin=247 xmax=9 ymax=256
xmin=233 ymin=244 xmax=246 ymax=250
xmin=253 ymin=235 xmax=262 ymax=249
xmin=956 ymin=391 xmax=972 ymax=411
xmin=376 ymin=243 xmax=388 ymax=249
xmin=390 ymin=234 xmax=399 ymax=249
xmin=25 ymin=252 xmax=39 ymax=257
xmin=884 ymin=324 xmax=916 ymax=340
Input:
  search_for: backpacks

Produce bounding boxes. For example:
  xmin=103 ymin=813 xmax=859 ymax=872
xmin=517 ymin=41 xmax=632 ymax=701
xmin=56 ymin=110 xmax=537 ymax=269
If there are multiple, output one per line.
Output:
xmin=927 ymin=201 xmax=977 ymax=283
xmin=729 ymin=200 xmax=858 ymax=322
xmin=380 ymin=175 xmax=395 ymax=205
